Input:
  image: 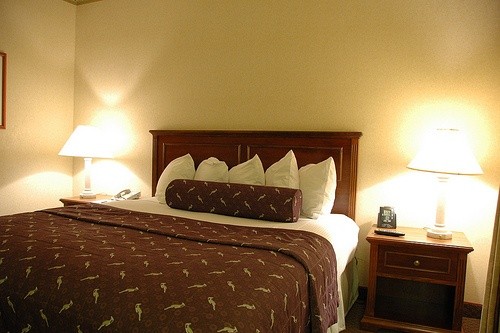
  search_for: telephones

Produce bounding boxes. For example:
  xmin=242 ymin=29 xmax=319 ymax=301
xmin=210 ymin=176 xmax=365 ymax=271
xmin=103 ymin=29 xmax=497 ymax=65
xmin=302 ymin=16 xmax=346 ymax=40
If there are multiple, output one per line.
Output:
xmin=111 ymin=189 xmax=141 ymax=201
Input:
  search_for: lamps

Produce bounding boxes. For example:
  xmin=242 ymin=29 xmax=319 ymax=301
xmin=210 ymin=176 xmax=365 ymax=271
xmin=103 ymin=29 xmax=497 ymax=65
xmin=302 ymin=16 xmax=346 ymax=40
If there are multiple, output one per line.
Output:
xmin=405 ymin=128 xmax=484 ymax=240
xmin=58 ymin=125 xmax=113 ymax=199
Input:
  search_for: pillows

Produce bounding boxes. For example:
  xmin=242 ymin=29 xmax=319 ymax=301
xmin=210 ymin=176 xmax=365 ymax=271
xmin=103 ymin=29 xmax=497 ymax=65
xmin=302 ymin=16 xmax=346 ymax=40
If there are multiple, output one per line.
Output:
xmin=155 ymin=154 xmax=195 ymax=204
xmin=265 ymin=149 xmax=300 ymax=188
xmin=165 ymin=179 xmax=302 ymax=223
xmin=299 ymin=157 xmax=338 ymax=219
xmin=228 ymin=154 xmax=265 ymax=185
xmin=194 ymin=156 xmax=228 ymax=182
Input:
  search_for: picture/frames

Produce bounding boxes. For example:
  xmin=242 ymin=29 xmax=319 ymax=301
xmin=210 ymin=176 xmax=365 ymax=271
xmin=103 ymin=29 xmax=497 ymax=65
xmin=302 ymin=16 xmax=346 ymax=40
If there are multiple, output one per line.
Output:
xmin=0 ymin=51 xmax=8 ymax=129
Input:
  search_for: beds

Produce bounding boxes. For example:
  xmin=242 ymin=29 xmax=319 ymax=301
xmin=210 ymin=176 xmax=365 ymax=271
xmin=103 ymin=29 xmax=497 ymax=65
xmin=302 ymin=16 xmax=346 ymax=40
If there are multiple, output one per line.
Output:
xmin=0 ymin=129 xmax=363 ymax=333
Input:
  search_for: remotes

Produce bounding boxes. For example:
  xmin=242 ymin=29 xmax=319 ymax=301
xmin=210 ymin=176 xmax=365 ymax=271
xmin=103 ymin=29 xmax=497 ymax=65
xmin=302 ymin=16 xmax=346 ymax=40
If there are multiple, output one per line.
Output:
xmin=375 ymin=229 xmax=405 ymax=236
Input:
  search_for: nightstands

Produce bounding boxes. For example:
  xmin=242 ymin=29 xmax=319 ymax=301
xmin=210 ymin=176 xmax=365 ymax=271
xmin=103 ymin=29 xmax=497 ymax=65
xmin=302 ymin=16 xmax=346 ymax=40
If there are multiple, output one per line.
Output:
xmin=59 ymin=194 xmax=112 ymax=206
xmin=360 ymin=224 xmax=474 ymax=333
